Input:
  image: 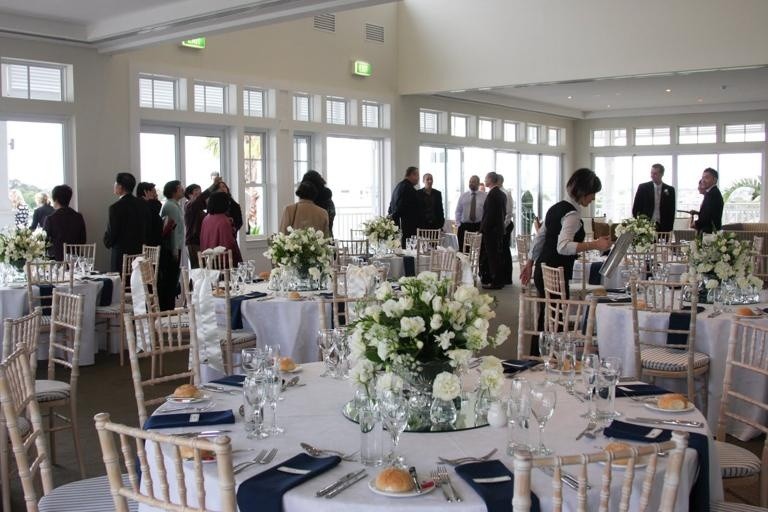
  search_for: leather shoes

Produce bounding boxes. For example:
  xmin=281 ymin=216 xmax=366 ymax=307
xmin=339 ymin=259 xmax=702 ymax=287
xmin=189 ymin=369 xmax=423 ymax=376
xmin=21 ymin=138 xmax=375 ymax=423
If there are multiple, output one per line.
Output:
xmin=482 ymin=283 xmax=505 ymax=290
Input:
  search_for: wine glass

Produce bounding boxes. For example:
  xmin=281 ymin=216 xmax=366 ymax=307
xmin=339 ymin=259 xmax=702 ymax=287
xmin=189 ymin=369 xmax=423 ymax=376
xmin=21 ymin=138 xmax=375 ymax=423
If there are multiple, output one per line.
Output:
xmin=351 ymin=235 xmax=435 ymax=258
xmin=617 ymin=261 xmax=762 ymax=306
xmin=578 ymin=236 xmax=691 ymax=264
xmin=233 ymin=318 xmax=626 ymax=467
xmin=1 ymin=252 xmax=95 ymax=290
xmin=217 ymin=258 xmax=394 ymax=305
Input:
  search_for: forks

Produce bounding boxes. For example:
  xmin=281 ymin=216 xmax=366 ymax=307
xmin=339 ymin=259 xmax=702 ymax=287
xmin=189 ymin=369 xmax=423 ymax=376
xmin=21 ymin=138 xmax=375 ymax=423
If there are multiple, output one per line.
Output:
xmin=152 ymin=357 xmax=705 ymax=503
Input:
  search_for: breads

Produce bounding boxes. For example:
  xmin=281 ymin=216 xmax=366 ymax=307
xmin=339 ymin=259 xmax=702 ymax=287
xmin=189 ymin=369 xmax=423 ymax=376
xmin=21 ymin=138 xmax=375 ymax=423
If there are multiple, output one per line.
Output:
xmin=594 ymin=289 xmax=607 ymax=296
xmin=637 ymin=300 xmax=647 ymax=308
xmin=658 ymin=394 xmax=689 ymax=410
xmin=607 ymin=442 xmax=640 ymax=466
xmin=174 ymin=384 xmax=200 ymax=402
xmin=280 ymin=357 xmax=296 ymax=371
xmin=377 ymin=468 xmax=414 ymax=493
xmin=737 ymin=308 xmax=753 ymax=316
xmin=179 ymin=438 xmax=208 ymax=459
xmin=288 ymin=291 xmax=301 ymax=299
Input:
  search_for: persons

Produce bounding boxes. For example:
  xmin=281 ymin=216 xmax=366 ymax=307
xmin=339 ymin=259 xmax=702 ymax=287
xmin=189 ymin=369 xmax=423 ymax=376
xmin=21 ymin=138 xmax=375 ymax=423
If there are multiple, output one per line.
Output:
xmin=100 ymin=170 xmax=243 ymax=334
xmin=276 ymin=180 xmax=330 ymax=238
xmin=40 ymin=184 xmax=88 ymax=261
xmin=518 ymin=167 xmax=613 ymax=354
xmin=690 ymin=180 xmax=707 ymax=216
xmin=8 ymin=188 xmax=30 ymax=230
xmin=384 ymin=165 xmax=514 ymax=292
xmin=28 ymin=192 xmax=55 ymax=233
xmin=689 ymin=166 xmax=724 ymax=232
xmin=631 ymin=163 xmax=675 ymax=243
xmin=302 ymin=170 xmax=338 ymax=260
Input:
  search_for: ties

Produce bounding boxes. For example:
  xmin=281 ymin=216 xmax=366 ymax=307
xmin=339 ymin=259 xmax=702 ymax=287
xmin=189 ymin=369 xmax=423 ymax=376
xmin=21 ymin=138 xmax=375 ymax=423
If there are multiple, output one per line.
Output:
xmin=469 ymin=194 xmax=478 ymax=222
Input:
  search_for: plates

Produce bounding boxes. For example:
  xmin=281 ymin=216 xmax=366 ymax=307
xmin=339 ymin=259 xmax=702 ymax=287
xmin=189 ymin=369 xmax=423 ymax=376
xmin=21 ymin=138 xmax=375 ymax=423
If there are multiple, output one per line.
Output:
xmin=587 ymin=285 xmax=768 ymax=322
